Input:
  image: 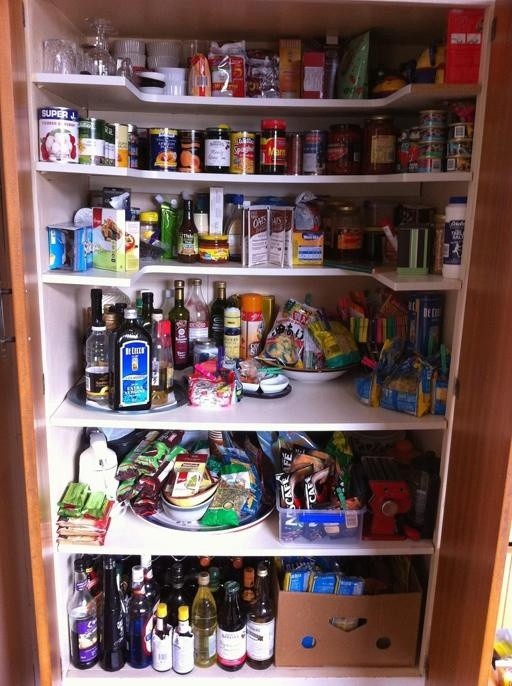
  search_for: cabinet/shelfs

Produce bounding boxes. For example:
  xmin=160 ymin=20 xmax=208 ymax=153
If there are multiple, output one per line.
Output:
xmin=15 ymin=0 xmax=496 ymax=679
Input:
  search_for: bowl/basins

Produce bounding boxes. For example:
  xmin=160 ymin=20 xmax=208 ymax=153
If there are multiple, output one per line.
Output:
xmin=160 ymin=490 xmax=214 ymax=522
xmin=113 ymin=38 xmax=182 ymax=70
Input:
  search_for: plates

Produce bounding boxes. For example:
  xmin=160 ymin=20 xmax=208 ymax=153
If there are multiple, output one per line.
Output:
xmin=242 ymin=374 xmax=290 ymax=393
xmin=127 ymin=501 xmax=275 ymax=535
xmin=266 ymin=363 xmax=348 ymax=383
xmin=66 ymin=382 xmax=186 ymax=414
xmin=244 ymin=385 xmax=292 ymax=399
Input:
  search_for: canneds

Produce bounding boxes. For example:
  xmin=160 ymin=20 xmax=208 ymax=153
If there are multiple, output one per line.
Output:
xmin=445 ymin=122 xmax=475 ymax=140
xmin=176 ymin=129 xmax=206 ymax=173
xmin=418 ymin=108 xmax=447 ymax=129
xmin=300 ymin=129 xmax=327 ymax=175
xmin=396 ymin=140 xmax=409 ymax=174
xmin=322 ymin=122 xmax=364 ymax=176
xmin=228 ymin=129 xmax=258 ymax=174
xmin=285 ymin=131 xmax=304 ymax=175
xmin=441 ymin=155 xmax=471 ymax=174
xmin=408 ymin=139 xmax=421 ymax=174
xmin=359 ymin=113 xmax=397 ymax=175
xmin=147 ymin=127 xmax=179 ymax=173
xmin=417 ymin=141 xmax=443 ymax=158
xmin=202 ymin=127 xmax=231 ymax=174
xmin=257 ymin=118 xmax=288 ymax=175
xmin=442 ymin=138 xmax=473 ymax=157
xmin=416 ymin=158 xmax=442 ymax=174
xmin=198 ymin=233 xmax=231 ymax=265
xmin=397 ymin=126 xmax=421 ymax=142
xmin=417 ymin=129 xmax=445 ymax=144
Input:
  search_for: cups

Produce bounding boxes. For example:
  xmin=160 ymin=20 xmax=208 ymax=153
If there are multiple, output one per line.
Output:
xmin=140 ymin=72 xmax=165 ymax=94
xmin=44 ymin=39 xmax=77 ymax=75
xmin=156 ymin=68 xmax=186 ymax=94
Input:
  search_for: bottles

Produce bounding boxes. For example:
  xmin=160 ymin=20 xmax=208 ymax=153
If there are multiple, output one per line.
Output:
xmin=434 ymin=196 xmax=467 ymax=278
xmin=225 ymin=194 xmax=244 ymax=259
xmin=178 ymin=201 xmax=208 ymax=264
xmin=69 ymin=555 xmax=275 ymax=675
xmin=81 ymin=279 xmax=225 ymax=412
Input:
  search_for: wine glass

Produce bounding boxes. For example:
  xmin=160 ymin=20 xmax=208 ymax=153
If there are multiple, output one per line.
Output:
xmin=84 ymin=16 xmax=117 ymax=76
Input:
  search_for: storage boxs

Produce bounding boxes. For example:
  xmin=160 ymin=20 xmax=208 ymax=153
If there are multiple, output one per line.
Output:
xmin=276 ymin=486 xmax=366 ymax=547
xmin=271 ymin=565 xmax=422 ymax=666
